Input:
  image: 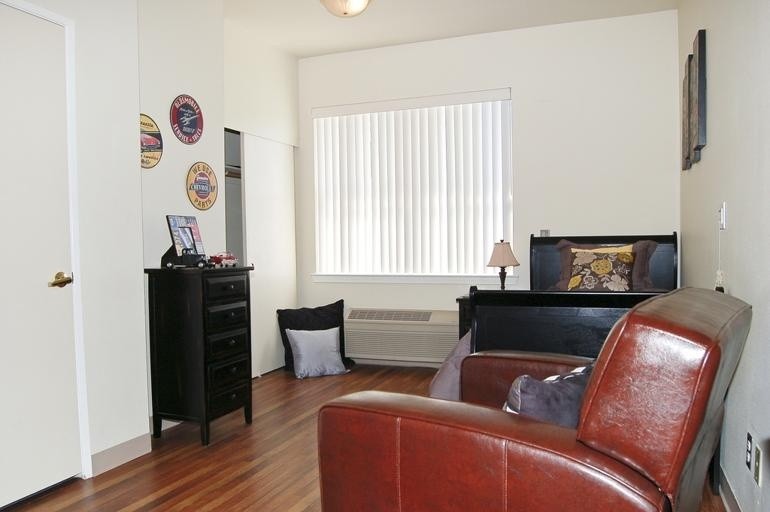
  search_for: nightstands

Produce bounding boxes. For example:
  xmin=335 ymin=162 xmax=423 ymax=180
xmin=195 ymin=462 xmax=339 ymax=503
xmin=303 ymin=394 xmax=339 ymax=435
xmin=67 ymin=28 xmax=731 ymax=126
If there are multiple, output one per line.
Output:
xmin=455 ymin=295 xmax=473 ymax=338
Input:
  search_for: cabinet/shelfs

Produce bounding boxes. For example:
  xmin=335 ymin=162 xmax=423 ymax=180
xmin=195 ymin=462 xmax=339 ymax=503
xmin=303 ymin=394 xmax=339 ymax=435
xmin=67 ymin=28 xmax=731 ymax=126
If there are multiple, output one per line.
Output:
xmin=143 ymin=266 xmax=254 ymax=441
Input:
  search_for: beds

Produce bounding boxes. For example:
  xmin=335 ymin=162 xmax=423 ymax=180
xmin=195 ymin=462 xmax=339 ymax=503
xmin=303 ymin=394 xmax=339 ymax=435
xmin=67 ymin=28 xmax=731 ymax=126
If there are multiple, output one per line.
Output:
xmin=459 ymin=233 xmax=678 ymax=357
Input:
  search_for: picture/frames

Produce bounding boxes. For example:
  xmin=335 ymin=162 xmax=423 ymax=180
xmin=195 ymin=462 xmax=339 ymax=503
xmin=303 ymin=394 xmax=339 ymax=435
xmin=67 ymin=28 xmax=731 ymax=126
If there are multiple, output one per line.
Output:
xmin=681 ymin=31 xmax=707 ymax=173
xmin=166 ymin=215 xmax=207 ymax=266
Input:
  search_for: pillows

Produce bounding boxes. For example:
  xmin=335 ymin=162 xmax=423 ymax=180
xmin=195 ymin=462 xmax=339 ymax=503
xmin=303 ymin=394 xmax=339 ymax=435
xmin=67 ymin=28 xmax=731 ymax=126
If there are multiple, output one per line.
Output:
xmin=503 ymin=363 xmax=600 ymax=429
xmin=569 ymin=250 xmax=634 ymax=293
xmin=553 ymin=239 xmax=657 ymax=290
xmin=274 ymin=299 xmax=356 ymax=374
xmin=285 ymin=327 xmax=350 ymax=379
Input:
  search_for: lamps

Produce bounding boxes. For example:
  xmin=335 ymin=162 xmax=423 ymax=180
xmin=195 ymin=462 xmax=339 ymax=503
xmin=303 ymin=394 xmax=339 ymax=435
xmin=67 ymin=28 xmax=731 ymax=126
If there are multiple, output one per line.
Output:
xmin=490 ymin=239 xmax=517 ymax=288
xmin=319 ymin=1 xmax=372 ymax=18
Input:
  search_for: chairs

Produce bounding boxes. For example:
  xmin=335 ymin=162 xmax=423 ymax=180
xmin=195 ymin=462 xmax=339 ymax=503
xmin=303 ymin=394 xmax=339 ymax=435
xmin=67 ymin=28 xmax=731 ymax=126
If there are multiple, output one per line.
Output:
xmin=316 ymin=287 xmax=755 ymax=512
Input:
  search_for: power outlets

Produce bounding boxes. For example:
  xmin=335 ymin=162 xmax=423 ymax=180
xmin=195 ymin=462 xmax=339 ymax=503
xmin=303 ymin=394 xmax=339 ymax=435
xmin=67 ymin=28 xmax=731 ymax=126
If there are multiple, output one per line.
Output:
xmin=745 ymin=432 xmax=753 ymax=472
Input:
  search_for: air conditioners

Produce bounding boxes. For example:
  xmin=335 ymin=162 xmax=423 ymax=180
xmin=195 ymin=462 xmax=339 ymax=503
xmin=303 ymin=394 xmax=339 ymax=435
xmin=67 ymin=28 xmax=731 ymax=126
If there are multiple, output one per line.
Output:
xmin=340 ymin=304 xmax=462 ymax=367
